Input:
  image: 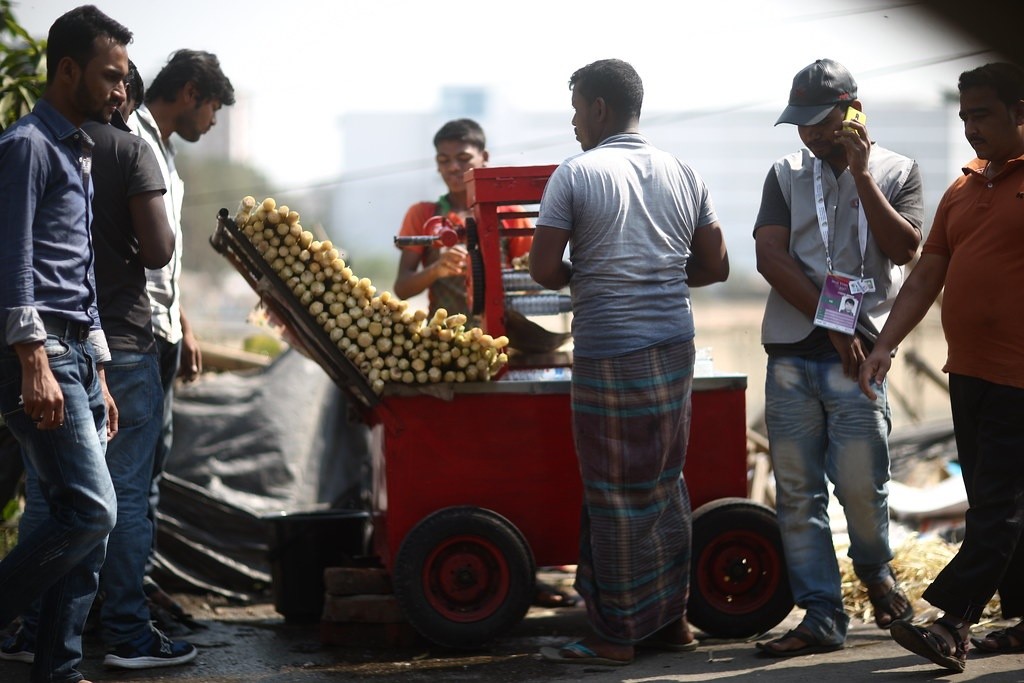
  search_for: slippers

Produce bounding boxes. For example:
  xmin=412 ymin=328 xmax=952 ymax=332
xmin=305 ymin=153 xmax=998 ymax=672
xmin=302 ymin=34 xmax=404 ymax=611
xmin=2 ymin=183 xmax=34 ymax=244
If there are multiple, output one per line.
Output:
xmin=636 ymin=638 xmax=699 ymax=652
xmin=540 ymin=641 xmax=633 ymax=666
xmin=754 ymin=630 xmax=843 ymax=657
xmin=867 ymin=562 xmax=914 ymax=629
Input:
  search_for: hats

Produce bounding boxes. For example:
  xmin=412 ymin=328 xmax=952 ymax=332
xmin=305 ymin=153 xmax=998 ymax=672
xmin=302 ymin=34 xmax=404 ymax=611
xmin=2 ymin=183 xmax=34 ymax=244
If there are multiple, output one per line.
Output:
xmin=774 ymin=59 xmax=858 ymax=127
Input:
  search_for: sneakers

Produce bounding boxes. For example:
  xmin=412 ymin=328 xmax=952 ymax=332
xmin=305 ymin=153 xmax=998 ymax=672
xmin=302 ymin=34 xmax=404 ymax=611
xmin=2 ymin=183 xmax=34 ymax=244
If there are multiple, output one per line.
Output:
xmin=0 ymin=625 xmax=36 ymax=663
xmin=102 ymin=619 xmax=197 ymax=668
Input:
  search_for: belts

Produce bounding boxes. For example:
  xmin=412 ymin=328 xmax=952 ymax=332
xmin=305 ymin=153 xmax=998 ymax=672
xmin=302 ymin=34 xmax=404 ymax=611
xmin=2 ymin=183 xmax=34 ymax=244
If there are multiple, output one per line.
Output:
xmin=39 ymin=314 xmax=89 ymax=342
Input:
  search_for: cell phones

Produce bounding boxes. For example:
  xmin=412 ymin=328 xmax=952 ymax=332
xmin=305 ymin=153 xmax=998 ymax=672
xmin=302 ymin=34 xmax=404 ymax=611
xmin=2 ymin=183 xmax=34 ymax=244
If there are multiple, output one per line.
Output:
xmin=843 ymin=106 xmax=866 ymax=143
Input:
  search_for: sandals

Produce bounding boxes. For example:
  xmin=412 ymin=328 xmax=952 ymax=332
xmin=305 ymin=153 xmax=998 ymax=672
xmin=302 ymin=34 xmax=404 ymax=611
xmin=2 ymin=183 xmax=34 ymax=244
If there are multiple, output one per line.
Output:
xmin=970 ymin=623 xmax=1024 ymax=655
xmin=889 ymin=617 xmax=969 ymax=672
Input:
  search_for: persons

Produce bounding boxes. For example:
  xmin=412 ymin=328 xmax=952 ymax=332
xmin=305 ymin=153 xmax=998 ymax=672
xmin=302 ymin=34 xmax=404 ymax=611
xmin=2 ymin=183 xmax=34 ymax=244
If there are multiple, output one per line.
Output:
xmin=858 ymin=61 xmax=1024 ymax=673
xmin=393 ymin=118 xmax=534 ymax=332
xmin=0 ymin=6 xmax=236 ymax=683
xmin=751 ymin=58 xmax=924 ymax=656
xmin=841 ymin=299 xmax=854 ymax=317
xmin=528 ymin=59 xmax=729 ymax=664
